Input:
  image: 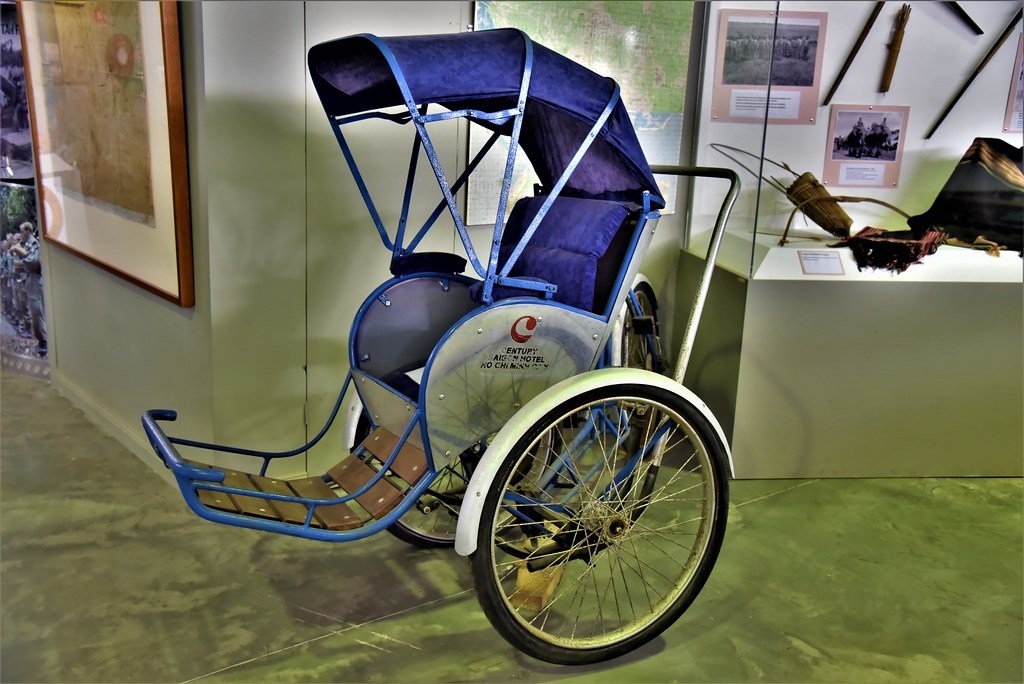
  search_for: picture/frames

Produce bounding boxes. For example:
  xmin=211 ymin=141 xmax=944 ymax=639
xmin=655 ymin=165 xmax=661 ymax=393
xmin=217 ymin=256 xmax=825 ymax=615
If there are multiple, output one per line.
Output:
xmin=15 ymin=0 xmax=196 ymax=308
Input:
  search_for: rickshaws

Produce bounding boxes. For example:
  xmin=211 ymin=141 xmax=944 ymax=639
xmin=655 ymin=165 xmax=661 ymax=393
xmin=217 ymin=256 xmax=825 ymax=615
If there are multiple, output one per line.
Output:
xmin=137 ymin=29 xmax=743 ymax=666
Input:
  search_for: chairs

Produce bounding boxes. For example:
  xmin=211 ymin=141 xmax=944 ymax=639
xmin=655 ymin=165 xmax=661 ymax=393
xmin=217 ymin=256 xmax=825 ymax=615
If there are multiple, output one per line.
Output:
xmin=349 ymin=192 xmax=631 ymax=472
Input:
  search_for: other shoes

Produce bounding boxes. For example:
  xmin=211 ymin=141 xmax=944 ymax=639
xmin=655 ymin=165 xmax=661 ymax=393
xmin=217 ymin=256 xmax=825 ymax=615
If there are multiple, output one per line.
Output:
xmin=1 ymin=300 xmax=47 ymax=356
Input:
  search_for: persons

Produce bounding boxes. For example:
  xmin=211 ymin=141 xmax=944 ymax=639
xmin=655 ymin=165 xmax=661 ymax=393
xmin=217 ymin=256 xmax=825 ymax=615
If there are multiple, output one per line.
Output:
xmin=725 ymin=33 xmax=810 ymax=61
xmin=1 ymin=220 xmax=47 ymax=357
xmin=837 ymin=117 xmax=892 ymax=158
